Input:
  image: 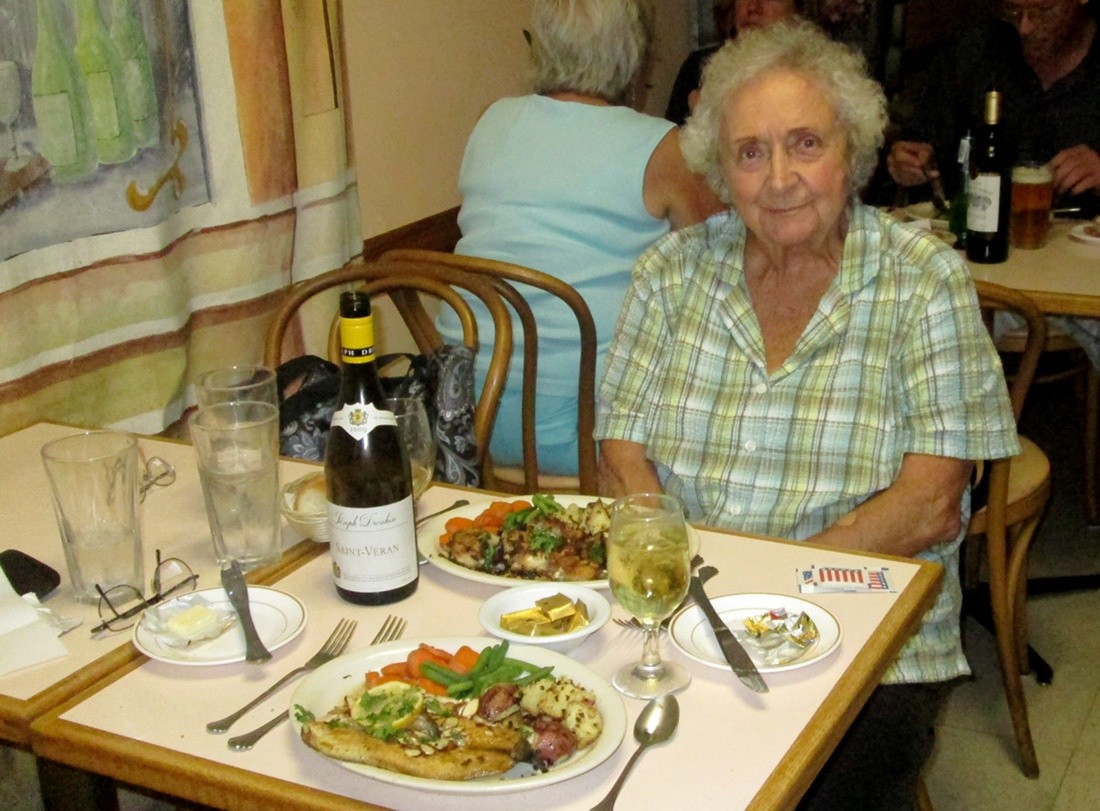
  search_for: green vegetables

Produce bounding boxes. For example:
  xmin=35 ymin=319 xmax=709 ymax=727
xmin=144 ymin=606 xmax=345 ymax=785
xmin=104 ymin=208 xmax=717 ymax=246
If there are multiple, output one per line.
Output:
xmin=292 ymin=686 xmax=465 ymax=750
xmin=483 ymin=492 xmax=606 ymax=570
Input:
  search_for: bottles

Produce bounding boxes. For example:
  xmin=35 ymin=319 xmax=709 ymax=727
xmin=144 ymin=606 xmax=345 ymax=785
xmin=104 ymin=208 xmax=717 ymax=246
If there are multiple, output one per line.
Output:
xmin=326 ymin=291 xmax=421 ymax=606
xmin=965 ymin=89 xmax=1014 ymax=263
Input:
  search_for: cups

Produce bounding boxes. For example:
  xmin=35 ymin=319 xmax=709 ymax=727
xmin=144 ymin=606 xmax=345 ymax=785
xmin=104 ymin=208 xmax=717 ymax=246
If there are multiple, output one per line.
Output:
xmin=388 ymin=398 xmax=435 ymax=499
xmin=40 ymin=429 xmax=146 ymax=608
xmin=189 ymin=400 xmax=283 ymax=571
xmin=1008 ymin=162 xmax=1055 ymax=252
xmin=192 ymin=363 xmax=281 ymax=416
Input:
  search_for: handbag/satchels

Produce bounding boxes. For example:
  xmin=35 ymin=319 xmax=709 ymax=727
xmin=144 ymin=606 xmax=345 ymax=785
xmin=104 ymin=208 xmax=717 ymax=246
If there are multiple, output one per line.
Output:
xmin=271 ymin=347 xmax=480 ymax=492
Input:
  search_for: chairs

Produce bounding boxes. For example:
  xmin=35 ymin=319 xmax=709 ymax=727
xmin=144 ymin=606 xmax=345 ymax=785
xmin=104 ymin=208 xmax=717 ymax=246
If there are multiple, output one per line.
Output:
xmin=989 ymin=320 xmax=1100 ymax=528
xmin=966 ymin=277 xmax=1059 ymax=777
xmin=376 ymin=248 xmax=597 ymax=496
xmin=267 ymin=262 xmax=536 ymax=494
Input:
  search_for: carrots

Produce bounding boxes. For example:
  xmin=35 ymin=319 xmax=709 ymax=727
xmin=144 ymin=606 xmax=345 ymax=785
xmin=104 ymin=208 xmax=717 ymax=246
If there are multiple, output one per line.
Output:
xmin=439 ymin=501 xmax=533 ymax=550
xmin=362 ymin=643 xmax=480 ymax=696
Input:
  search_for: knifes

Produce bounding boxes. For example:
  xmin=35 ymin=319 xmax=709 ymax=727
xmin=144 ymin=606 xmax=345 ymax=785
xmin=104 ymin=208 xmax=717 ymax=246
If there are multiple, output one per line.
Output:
xmin=921 ymin=166 xmax=948 ymax=216
xmin=689 ymin=573 xmax=769 ymax=693
xmin=220 ymin=560 xmax=273 ymax=665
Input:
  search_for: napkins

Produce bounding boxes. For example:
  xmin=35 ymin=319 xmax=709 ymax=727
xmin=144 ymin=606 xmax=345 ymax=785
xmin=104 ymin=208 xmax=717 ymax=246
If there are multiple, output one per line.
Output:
xmin=0 ymin=589 xmax=82 ymax=676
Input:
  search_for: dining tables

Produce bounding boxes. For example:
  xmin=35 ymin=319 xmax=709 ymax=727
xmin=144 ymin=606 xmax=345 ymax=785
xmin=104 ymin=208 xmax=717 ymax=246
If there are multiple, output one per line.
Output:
xmin=904 ymin=203 xmax=1100 ymax=317
xmin=28 ymin=483 xmax=946 ymax=811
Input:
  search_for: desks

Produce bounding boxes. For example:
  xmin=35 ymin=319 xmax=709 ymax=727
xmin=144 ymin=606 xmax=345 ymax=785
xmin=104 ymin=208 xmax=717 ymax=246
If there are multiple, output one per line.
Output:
xmin=0 ymin=417 xmax=326 ymax=749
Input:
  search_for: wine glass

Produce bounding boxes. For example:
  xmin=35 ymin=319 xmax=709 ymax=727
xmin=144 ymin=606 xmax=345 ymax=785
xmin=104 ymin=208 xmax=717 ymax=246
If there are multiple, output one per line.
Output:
xmin=605 ymin=492 xmax=692 ymax=699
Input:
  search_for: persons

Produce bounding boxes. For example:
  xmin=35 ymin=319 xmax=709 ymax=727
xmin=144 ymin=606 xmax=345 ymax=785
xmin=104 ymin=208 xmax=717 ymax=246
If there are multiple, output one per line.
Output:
xmin=593 ymin=20 xmax=1020 ymax=811
xmin=665 ymin=0 xmax=826 ymax=125
xmin=435 ymin=0 xmax=727 ymax=476
xmin=886 ymin=0 xmax=1100 ymax=221
xmin=820 ymin=0 xmax=885 ymax=57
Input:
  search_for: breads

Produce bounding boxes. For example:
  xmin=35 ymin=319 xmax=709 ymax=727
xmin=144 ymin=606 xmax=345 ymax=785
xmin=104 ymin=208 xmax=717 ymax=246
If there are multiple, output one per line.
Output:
xmin=293 ymin=475 xmax=327 ymax=513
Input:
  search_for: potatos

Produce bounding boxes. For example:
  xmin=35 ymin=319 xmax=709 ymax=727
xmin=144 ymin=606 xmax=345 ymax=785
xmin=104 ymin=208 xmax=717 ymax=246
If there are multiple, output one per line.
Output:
xmin=476 ymin=678 xmax=603 ymax=769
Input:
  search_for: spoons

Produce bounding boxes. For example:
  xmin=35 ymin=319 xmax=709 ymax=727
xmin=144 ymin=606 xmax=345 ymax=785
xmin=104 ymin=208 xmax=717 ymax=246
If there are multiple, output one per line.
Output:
xmin=589 ymin=692 xmax=679 ymax=810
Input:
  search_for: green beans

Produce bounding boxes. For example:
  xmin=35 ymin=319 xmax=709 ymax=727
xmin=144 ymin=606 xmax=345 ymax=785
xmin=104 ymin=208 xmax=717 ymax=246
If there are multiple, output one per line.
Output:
xmin=416 ymin=638 xmax=557 ymax=700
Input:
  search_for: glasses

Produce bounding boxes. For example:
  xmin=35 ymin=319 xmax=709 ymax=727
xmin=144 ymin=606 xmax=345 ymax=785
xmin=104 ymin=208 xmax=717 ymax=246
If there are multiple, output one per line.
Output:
xmin=999 ymin=0 xmax=1071 ymax=23
xmin=108 ymin=452 xmax=176 ymax=507
xmin=91 ymin=548 xmax=201 ymax=634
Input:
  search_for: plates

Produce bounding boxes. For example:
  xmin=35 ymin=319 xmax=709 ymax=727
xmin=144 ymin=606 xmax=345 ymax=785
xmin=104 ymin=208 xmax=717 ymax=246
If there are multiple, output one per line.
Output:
xmin=668 ymin=593 xmax=844 ymax=673
xmin=131 ymin=584 xmax=307 ymax=667
xmin=904 ymin=200 xmax=952 ymax=231
xmin=289 ymin=635 xmax=629 ymax=794
xmin=416 ymin=494 xmax=702 ymax=591
xmin=1069 ymin=222 xmax=1100 ymax=242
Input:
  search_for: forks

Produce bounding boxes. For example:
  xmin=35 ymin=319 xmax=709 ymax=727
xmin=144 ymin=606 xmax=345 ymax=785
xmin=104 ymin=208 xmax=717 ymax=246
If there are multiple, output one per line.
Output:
xmin=206 ymin=618 xmax=358 ymax=734
xmin=226 ymin=615 xmax=406 ymax=751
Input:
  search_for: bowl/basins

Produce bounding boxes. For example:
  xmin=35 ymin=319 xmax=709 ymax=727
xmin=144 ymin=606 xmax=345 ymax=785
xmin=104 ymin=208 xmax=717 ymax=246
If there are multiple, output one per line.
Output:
xmin=477 ymin=581 xmax=613 ymax=653
xmin=934 ymin=230 xmax=957 ymax=247
xmin=280 ymin=461 xmax=428 ymax=543
